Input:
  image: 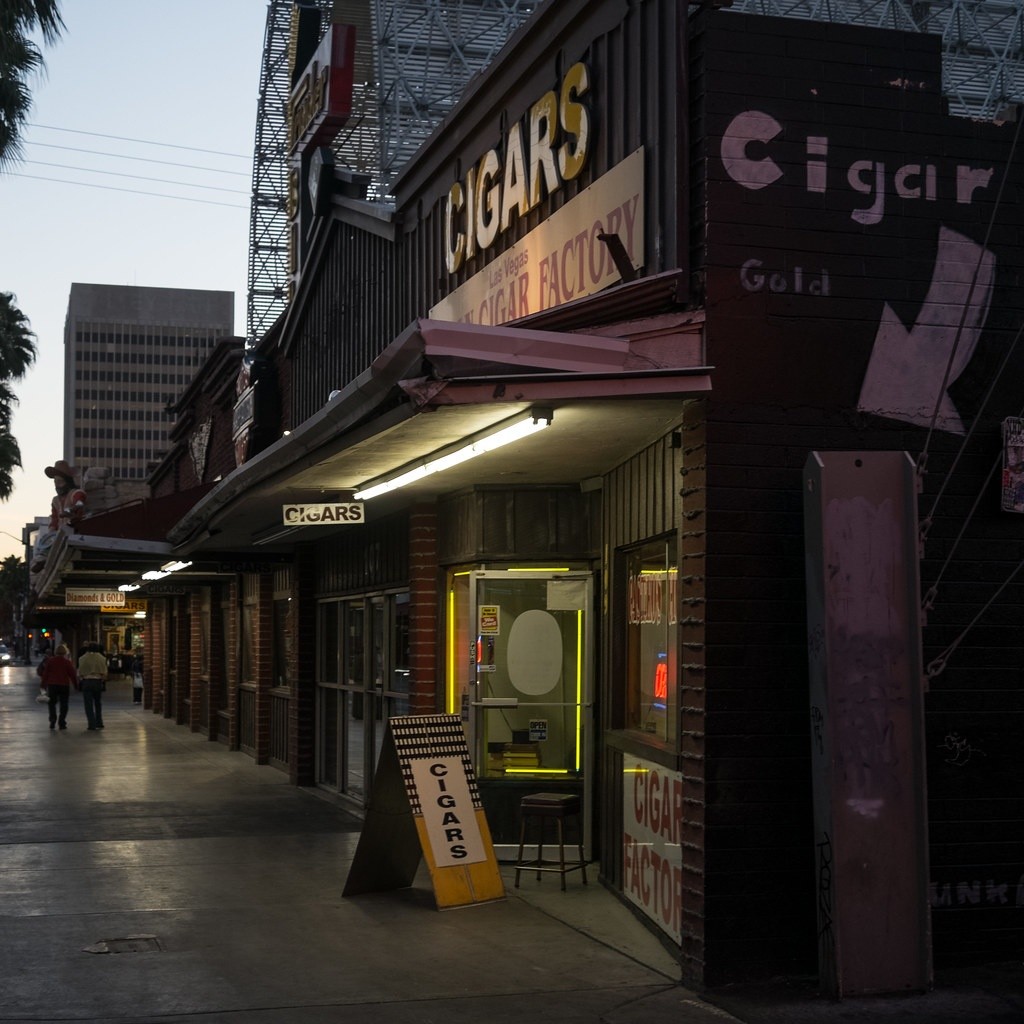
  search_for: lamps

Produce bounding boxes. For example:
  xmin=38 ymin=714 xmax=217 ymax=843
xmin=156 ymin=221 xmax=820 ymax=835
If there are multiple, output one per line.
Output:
xmin=117 ymin=558 xmax=193 ymax=592
xmin=353 ymin=407 xmax=554 ymax=501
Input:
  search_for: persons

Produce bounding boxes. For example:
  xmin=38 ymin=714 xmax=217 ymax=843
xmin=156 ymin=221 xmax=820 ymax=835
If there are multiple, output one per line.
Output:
xmin=261 ymin=602 xmax=287 ymax=686
xmin=37 ymin=644 xmax=75 ymax=730
xmin=132 ymin=656 xmax=143 ymax=705
xmin=78 ymin=641 xmax=108 ymax=730
xmin=29 ymin=461 xmax=87 ymax=574
xmin=107 ymin=645 xmax=122 ymax=674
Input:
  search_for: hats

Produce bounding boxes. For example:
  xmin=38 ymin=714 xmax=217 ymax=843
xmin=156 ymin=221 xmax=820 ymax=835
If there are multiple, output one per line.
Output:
xmin=56 ymin=644 xmax=68 ymax=654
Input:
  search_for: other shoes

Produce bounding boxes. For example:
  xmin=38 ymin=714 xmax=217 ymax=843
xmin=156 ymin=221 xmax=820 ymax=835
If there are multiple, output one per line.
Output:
xmin=87 ymin=727 xmax=95 ymax=730
xmin=59 ymin=724 xmax=67 ymax=729
xmin=96 ymin=725 xmax=104 ymax=728
xmin=49 ymin=721 xmax=56 ymax=729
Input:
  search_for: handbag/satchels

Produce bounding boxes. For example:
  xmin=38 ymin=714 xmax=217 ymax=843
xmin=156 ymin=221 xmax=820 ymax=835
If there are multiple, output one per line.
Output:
xmin=35 ymin=687 xmax=50 ymax=704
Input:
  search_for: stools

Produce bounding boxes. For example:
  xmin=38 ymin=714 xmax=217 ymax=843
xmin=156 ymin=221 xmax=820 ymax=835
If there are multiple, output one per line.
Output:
xmin=513 ymin=792 xmax=591 ymax=894
